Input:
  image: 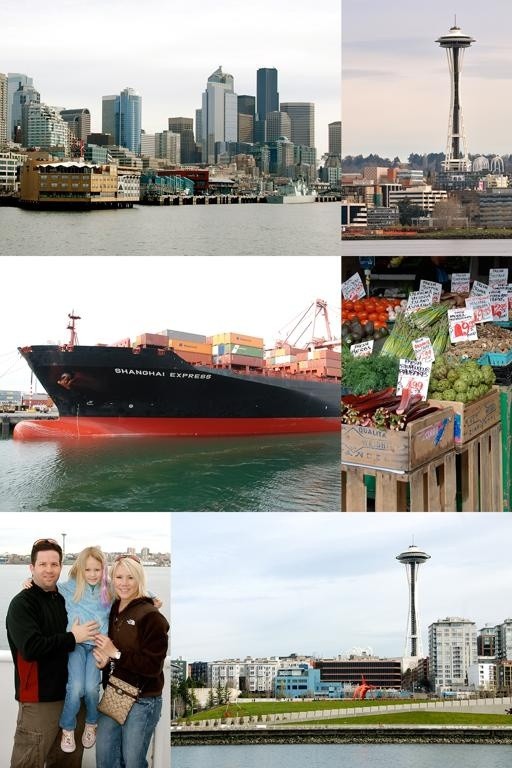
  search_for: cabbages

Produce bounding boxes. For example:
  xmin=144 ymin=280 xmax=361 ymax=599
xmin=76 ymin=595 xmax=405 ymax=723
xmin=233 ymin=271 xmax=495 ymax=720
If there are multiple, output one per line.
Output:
xmin=426 ymin=356 xmax=497 ymax=403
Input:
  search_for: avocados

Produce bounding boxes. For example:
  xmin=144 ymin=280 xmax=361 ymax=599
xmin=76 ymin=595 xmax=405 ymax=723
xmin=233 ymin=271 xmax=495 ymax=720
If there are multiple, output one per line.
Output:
xmin=341 ymin=316 xmax=388 ymax=345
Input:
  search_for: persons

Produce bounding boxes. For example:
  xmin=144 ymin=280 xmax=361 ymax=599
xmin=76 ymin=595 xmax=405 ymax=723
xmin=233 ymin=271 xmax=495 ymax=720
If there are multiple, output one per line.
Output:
xmin=91 ymin=553 xmax=173 ymax=768
xmin=4 ymin=536 xmax=88 ymax=768
xmin=22 ymin=543 xmax=165 ymax=757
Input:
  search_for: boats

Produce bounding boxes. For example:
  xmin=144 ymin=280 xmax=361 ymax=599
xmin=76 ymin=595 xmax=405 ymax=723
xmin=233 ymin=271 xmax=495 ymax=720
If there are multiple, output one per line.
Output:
xmin=266 ymin=177 xmax=316 ymax=204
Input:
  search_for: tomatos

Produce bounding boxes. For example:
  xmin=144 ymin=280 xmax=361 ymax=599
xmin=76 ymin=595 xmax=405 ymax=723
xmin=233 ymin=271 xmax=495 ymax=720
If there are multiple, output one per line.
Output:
xmin=342 ymin=296 xmax=401 ymax=329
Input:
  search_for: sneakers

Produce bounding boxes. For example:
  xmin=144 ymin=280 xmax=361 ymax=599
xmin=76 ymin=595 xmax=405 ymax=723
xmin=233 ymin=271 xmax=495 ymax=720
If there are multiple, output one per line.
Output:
xmin=82 ymin=723 xmax=98 ymax=748
xmin=61 ymin=729 xmax=76 ymax=753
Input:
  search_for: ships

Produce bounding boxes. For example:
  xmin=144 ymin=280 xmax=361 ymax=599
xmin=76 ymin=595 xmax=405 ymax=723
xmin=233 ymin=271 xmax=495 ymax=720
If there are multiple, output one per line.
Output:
xmin=14 ymin=308 xmax=341 ymax=440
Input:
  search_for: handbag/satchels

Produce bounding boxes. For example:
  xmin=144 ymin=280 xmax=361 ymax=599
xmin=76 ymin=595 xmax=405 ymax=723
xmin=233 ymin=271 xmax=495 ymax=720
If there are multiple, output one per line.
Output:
xmin=97 ymin=675 xmax=140 ymax=725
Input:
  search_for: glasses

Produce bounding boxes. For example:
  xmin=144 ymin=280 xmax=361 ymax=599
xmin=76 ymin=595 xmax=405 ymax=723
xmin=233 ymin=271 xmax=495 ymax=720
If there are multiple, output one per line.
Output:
xmin=114 ymin=555 xmax=140 ymax=563
xmin=34 ymin=539 xmax=57 ymax=545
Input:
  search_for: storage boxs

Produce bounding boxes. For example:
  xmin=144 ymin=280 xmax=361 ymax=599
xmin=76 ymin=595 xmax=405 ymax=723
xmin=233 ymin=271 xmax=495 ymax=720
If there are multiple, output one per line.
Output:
xmin=429 ymin=388 xmax=500 ymax=444
xmin=341 ymin=405 xmax=455 ymax=472
